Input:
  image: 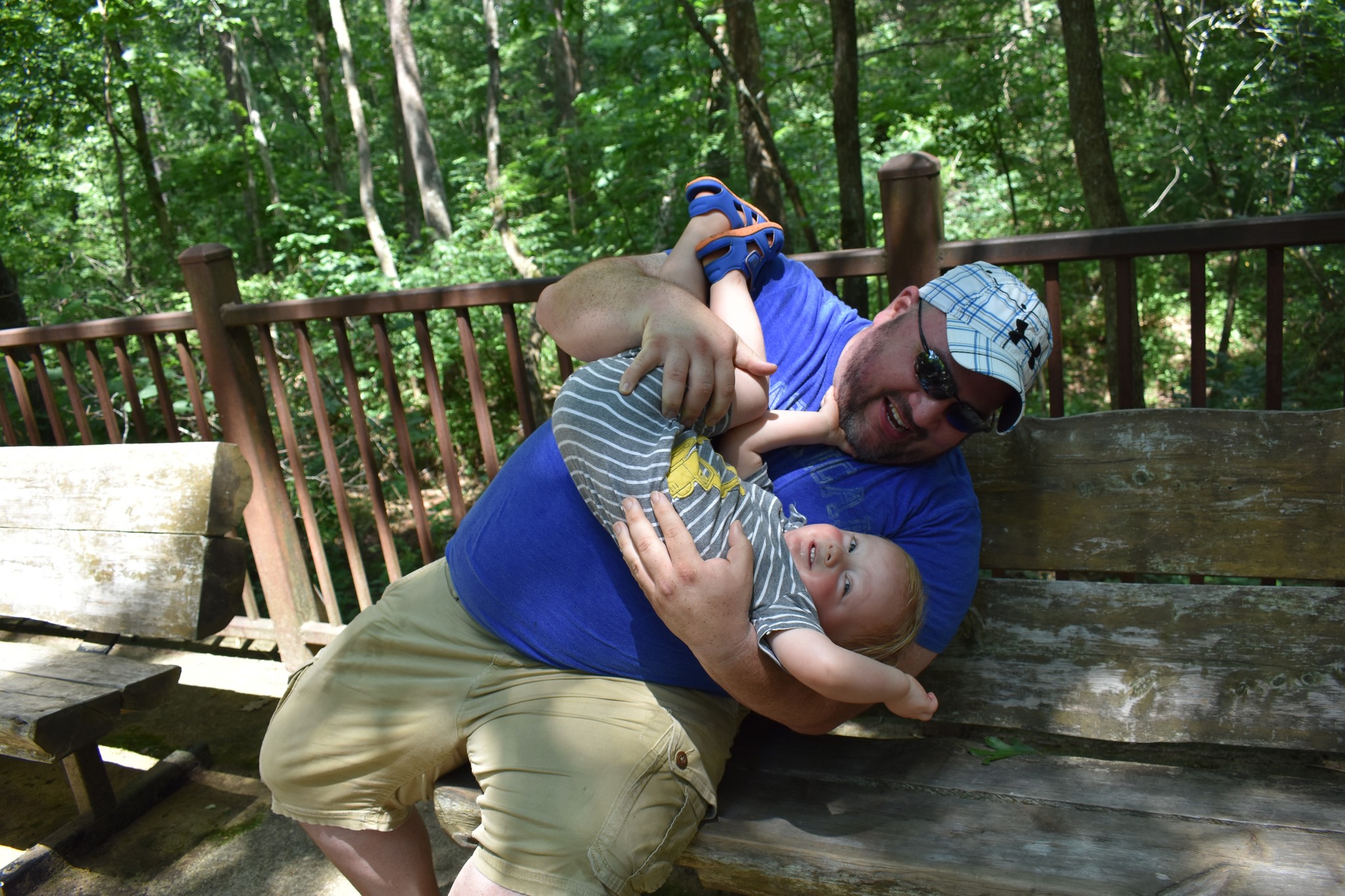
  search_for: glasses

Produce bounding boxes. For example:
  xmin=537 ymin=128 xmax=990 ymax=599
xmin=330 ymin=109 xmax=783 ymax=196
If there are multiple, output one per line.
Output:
xmin=913 ymin=297 xmax=1000 ymax=437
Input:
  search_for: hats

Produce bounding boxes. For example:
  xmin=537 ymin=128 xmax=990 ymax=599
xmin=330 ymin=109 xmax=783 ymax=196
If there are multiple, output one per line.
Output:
xmin=919 ymin=261 xmax=1054 ymax=435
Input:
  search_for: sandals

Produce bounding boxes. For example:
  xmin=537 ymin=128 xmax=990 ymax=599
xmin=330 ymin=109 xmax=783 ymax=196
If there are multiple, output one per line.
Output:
xmin=694 ymin=221 xmax=785 ymax=295
xmin=685 ymin=175 xmax=770 ymax=233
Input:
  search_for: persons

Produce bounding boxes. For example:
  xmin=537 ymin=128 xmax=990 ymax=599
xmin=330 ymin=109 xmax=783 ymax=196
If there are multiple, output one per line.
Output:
xmin=258 ymin=247 xmax=1055 ymax=896
xmin=550 ymin=173 xmax=940 ymax=722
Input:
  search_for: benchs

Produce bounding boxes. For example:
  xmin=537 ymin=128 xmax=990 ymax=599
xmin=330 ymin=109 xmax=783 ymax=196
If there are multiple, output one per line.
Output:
xmin=435 ymin=410 xmax=1345 ymax=896
xmin=0 ymin=441 xmax=256 ymax=896
